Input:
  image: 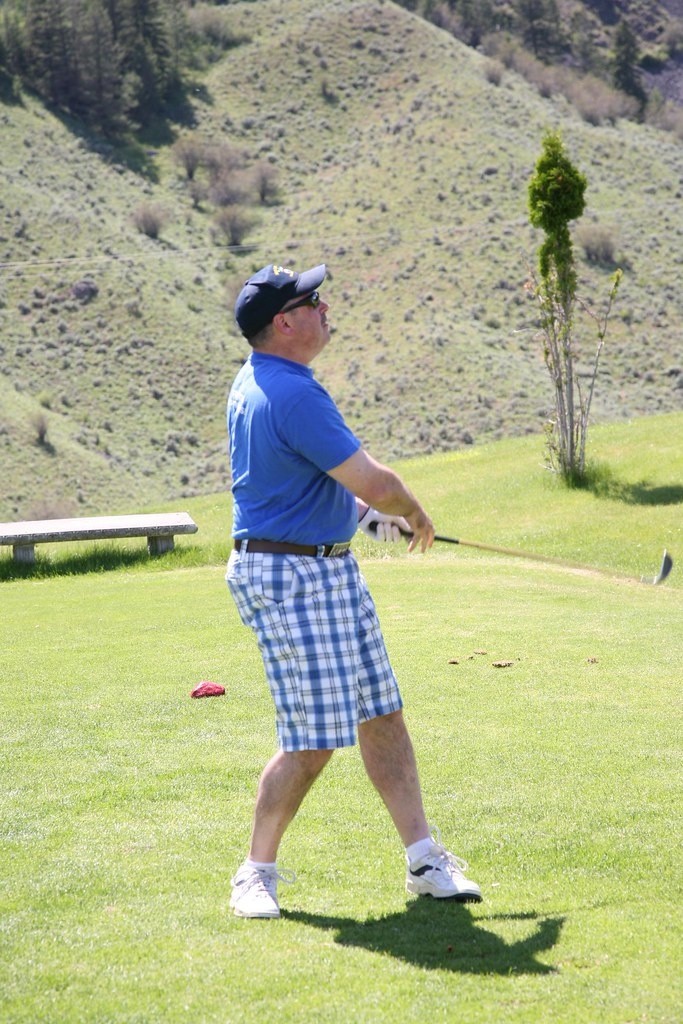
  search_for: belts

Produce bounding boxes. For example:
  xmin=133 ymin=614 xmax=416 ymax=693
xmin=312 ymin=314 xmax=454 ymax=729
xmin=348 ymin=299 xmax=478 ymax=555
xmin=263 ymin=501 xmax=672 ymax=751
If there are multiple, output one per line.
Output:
xmin=234 ymin=540 xmax=351 ymax=558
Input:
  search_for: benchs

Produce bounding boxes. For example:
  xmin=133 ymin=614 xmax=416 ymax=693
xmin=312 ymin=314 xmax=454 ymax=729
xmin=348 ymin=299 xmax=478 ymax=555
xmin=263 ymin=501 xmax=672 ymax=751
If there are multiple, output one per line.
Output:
xmin=0 ymin=511 xmax=199 ymax=568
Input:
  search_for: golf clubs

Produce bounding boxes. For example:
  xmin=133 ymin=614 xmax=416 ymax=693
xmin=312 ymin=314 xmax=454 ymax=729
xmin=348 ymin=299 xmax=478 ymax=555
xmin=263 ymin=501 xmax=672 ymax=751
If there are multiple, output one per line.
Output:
xmin=368 ymin=519 xmax=673 ymax=588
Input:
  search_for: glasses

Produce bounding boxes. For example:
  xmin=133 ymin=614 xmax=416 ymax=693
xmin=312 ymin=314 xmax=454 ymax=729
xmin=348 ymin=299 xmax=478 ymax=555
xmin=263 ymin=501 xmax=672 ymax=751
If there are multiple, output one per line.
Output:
xmin=281 ymin=291 xmax=321 ymax=315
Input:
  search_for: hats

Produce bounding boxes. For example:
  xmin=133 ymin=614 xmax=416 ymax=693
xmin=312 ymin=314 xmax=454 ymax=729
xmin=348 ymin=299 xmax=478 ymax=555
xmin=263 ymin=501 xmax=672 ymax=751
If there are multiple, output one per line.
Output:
xmin=234 ymin=264 xmax=325 ymax=339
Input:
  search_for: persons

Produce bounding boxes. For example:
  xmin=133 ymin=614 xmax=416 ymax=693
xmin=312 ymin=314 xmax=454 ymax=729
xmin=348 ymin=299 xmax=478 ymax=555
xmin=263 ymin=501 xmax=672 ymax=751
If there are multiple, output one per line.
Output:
xmin=225 ymin=263 xmax=483 ymax=919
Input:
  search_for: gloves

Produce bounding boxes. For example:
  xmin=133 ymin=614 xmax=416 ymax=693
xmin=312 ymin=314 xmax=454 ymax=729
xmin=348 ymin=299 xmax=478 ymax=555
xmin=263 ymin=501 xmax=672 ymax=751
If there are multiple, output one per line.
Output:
xmin=357 ymin=506 xmax=410 ymax=545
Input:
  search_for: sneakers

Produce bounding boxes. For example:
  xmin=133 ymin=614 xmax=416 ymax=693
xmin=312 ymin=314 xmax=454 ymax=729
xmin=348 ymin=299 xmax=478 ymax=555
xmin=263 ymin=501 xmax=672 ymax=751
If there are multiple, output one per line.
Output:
xmin=228 ymin=865 xmax=296 ymax=918
xmin=405 ymin=826 xmax=483 ymax=902
